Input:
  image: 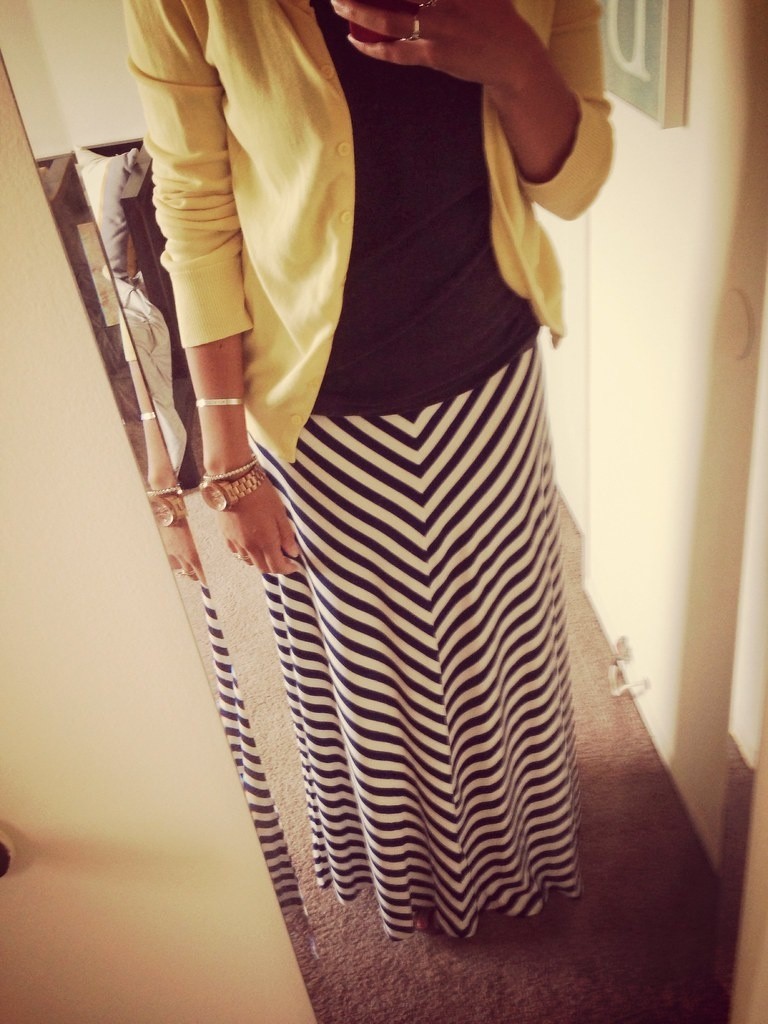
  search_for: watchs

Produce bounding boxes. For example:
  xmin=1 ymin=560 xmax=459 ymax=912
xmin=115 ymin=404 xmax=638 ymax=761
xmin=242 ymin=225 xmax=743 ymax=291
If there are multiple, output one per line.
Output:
xmin=149 ymin=496 xmax=185 ymax=529
xmin=198 ymin=464 xmax=265 ymax=513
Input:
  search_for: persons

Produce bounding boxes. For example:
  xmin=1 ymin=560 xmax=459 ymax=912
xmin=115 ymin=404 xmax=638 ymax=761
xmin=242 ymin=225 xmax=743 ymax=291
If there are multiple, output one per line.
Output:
xmin=117 ymin=304 xmax=301 ymax=910
xmin=122 ymin=0 xmax=613 ymax=945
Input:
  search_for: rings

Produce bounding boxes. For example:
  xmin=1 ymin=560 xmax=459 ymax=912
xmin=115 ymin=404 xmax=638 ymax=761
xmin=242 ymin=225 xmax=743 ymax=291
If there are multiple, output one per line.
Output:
xmin=398 ymin=13 xmax=421 ymax=42
xmin=175 ymin=566 xmax=195 ymax=578
xmin=418 ymin=0 xmax=437 ymax=9
xmin=233 ymin=551 xmax=250 ymax=563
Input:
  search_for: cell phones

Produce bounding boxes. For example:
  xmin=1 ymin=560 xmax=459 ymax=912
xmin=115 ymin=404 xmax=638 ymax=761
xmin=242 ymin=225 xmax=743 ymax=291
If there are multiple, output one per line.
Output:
xmin=348 ymin=0 xmax=419 ymax=45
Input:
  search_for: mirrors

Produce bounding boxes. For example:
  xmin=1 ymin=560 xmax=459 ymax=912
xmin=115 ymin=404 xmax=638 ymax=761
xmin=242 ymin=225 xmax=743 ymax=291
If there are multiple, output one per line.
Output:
xmin=0 ymin=0 xmax=767 ymax=1024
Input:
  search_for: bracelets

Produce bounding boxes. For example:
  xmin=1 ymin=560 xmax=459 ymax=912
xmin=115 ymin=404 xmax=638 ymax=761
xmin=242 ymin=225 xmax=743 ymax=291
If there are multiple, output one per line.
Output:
xmin=202 ymin=454 xmax=257 ymax=482
xmin=144 ymin=485 xmax=182 ymax=498
xmin=193 ymin=398 xmax=243 ymax=407
xmin=139 ymin=411 xmax=156 ymax=421
